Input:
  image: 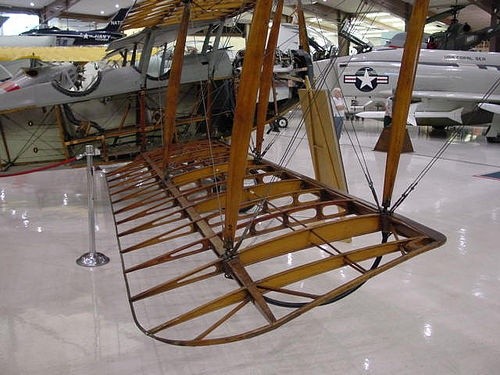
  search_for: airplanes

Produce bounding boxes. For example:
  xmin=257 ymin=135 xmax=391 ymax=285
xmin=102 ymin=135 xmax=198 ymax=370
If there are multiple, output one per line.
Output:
xmin=0 ymin=0 xmax=500 ymax=172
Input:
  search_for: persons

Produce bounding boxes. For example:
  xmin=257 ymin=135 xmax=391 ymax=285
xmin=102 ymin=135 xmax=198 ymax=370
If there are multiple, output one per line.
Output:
xmin=331 ymin=88 xmax=346 ymax=140
xmin=384 ymin=88 xmax=396 ymax=127
